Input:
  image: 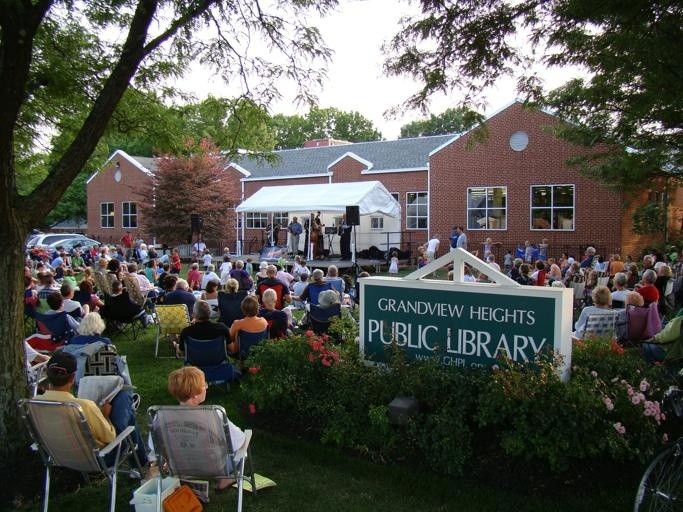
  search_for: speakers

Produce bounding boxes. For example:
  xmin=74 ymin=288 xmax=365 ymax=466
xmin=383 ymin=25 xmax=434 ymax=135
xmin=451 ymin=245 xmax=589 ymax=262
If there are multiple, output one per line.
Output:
xmin=191 ymin=214 xmax=204 ymax=232
xmin=346 ymin=206 xmax=360 ymax=226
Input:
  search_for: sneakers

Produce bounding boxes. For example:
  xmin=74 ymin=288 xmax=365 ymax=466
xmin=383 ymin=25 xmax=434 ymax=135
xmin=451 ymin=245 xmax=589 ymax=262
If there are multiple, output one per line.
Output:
xmin=131 ymin=393 xmax=141 ymax=412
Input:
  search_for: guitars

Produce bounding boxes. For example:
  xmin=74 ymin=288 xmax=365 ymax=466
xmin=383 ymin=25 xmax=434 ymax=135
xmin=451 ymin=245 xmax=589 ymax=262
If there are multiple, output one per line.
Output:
xmin=311 ymin=220 xmax=317 ymax=241
xmin=337 ymin=219 xmax=344 ymax=235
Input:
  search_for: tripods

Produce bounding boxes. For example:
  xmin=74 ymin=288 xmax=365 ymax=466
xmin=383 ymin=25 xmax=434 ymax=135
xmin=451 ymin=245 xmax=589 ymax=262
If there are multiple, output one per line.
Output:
xmin=344 ymin=225 xmax=364 ymax=276
xmin=193 ymin=233 xmax=202 ymax=263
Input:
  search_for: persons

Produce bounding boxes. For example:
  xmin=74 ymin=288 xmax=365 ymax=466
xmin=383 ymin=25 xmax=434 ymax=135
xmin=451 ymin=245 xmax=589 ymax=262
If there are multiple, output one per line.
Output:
xmin=148 ymin=366 xmax=248 ymax=493
xmin=25 ymin=341 xmax=51 ymax=393
xmin=32 ymin=352 xmax=148 ymax=479
xmin=24 ymin=212 xmax=673 ymax=361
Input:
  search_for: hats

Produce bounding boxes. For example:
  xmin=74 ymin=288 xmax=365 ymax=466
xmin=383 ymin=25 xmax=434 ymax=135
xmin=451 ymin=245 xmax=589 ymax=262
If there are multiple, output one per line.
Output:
xmin=47 ymin=352 xmax=77 ymax=375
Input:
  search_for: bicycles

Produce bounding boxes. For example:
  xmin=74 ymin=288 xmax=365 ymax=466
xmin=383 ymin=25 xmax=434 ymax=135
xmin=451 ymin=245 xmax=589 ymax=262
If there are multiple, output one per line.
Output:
xmin=633 ymin=429 xmax=682 ymax=512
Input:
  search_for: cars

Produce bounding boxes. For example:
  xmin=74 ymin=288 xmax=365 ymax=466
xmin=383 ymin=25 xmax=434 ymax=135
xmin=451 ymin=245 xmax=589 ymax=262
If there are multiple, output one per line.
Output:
xmin=26 ymin=233 xmax=101 ymax=253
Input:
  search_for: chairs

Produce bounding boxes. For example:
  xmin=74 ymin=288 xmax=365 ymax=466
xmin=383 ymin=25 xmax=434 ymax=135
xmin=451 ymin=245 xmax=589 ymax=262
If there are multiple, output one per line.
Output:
xmin=448 ymin=241 xmax=683 ymax=365
